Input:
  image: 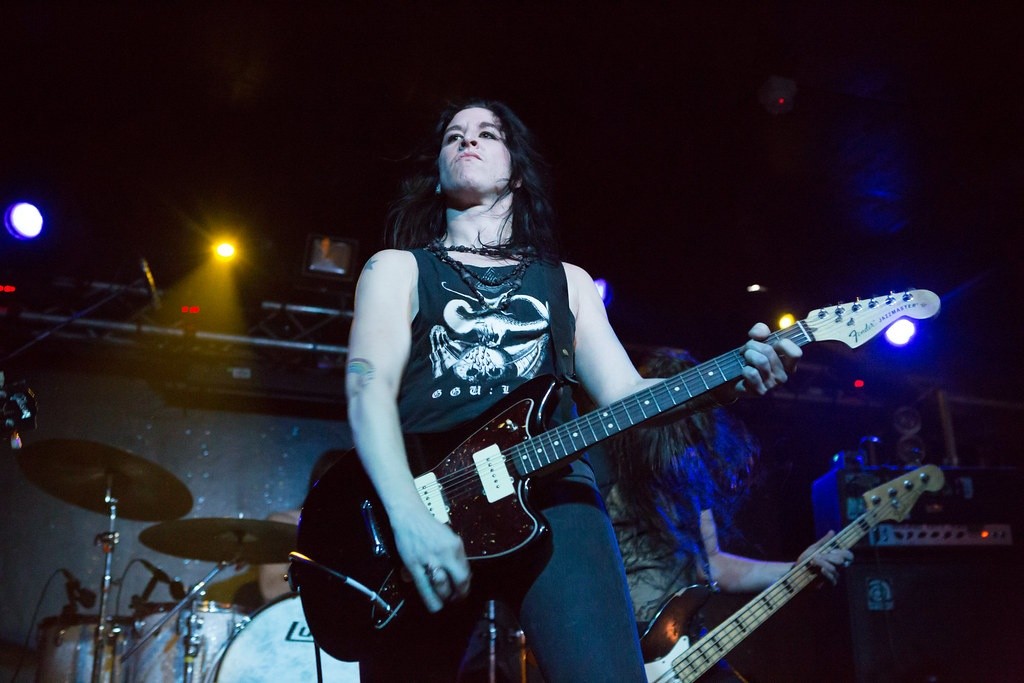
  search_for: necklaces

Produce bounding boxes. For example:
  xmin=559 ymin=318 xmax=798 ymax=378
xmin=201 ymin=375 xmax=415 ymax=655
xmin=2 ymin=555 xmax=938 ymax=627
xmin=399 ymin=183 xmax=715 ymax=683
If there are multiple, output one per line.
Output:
xmin=428 ymin=236 xmax=534 ymax=311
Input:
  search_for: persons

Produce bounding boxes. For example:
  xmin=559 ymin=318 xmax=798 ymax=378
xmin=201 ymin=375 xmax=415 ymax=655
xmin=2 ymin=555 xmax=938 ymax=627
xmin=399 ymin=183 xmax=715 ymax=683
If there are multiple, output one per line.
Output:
xmin=808 ymin=420 xmax=961 ymax=683
xmin=258 ymin=446 xmax=350 ymax=606
xmin=608 ymin=343 xmax=852 ymax=683
xmin=343 ymin=101 xmax=803 ymax=683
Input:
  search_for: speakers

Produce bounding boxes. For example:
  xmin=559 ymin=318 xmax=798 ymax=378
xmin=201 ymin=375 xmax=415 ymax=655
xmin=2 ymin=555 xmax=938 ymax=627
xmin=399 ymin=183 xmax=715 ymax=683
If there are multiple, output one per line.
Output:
xmin=844 ymin=544 xmax=1024 ymax=683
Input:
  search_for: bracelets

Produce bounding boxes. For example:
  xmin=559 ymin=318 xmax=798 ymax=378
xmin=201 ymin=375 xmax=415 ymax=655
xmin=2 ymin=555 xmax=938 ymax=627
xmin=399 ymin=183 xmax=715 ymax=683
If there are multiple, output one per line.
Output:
xmin=686 ymin=393 xmax=741 ymax=413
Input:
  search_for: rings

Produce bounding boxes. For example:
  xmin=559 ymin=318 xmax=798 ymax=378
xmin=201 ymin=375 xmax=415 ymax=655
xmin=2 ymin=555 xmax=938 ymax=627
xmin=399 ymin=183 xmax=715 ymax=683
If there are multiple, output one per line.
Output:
xmin=428 ymin=567 xmax=440 ymax=573
xmin=844 ymin=561 xmax=849 ymax=567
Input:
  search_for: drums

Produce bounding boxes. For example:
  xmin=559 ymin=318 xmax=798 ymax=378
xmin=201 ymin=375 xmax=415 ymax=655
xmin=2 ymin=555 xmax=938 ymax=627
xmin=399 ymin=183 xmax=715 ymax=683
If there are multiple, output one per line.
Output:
xmin=199 ymin=590 xmax=361 ymax=683
xmin=125 ymin=599 xmax=253 ymax=683
xmin=34 ymin=613 xmax=130 ymax=683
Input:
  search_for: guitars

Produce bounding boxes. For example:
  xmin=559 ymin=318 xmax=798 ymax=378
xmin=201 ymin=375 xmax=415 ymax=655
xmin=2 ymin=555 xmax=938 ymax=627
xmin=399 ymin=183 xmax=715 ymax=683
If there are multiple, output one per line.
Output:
xmin=282 ymin=288 xmax=937 ymax=665
xmin=639 ymin=462 xmax=947 ymax=683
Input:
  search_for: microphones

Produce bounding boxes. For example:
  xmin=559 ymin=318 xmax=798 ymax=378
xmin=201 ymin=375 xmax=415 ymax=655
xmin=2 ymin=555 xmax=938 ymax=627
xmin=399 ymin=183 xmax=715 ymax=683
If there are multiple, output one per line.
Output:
xmin=138 ymin=558 xmax=186 ymax=601
xmin=138 ymin=256 xmax=162 ymax=312
xmin=62 ymin=570 xmax=96 ymax=609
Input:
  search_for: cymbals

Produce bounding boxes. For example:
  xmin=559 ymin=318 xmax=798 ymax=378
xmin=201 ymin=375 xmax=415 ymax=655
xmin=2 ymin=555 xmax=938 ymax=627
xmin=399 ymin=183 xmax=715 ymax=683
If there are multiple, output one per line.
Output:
xmin=137 ymin=516 xmax=297 ymax=567
xmin=17 ymin=437 xmax=194 ymax=523
